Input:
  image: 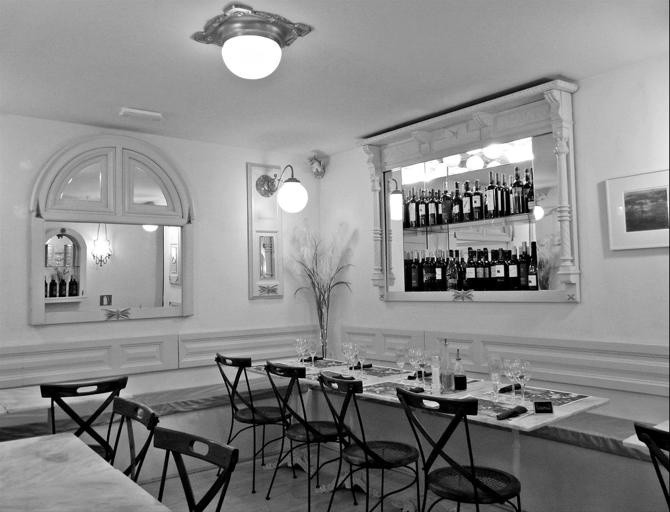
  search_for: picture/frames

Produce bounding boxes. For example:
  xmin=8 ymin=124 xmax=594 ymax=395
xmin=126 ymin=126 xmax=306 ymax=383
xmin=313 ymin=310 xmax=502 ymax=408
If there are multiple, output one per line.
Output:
xmin=606 ymin=168 xmax=670 ymax=250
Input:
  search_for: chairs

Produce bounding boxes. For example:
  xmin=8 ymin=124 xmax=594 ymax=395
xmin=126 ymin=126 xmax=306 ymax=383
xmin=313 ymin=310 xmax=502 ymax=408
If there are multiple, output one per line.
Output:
xmin=215 ymin=352 xmax=298 ymax=493
xmin=393 ymin=386 xmax=520 ymax=511
xmin=40 ymin=377 xmax=126 ymax=464
xmin=153 ymin=426 xmax=239 ymax=512
xmin=263 ymin=361 xmax=358 ymax=507
xmin=104 ymin=397 xmax=159 ymax=483
xmin=634 ymin=421 xmax=670 ymax=510
xmin=317 ymin=371 xmax=420 ymax=512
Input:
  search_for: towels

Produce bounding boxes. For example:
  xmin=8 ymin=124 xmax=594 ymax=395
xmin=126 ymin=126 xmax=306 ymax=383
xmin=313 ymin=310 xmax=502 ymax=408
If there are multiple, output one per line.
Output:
xmin=497 ymin=406 xmax=527 ymax=419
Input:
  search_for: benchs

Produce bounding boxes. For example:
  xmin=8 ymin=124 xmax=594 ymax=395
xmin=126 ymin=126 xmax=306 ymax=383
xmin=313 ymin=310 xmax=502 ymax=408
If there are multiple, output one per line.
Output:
xmin=1 ymin=328 xmax=317 ymax=441
xmin=343 ymin=325 xmax=670 ymax=465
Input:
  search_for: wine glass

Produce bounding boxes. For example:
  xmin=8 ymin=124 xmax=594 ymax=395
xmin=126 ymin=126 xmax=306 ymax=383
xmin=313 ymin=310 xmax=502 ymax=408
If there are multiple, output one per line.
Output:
xmin=339 ymin=341 xmax=368 ymax=381
xmin=294 ymin=333 xmax=318 ymax=373
xmin=487 ymin=356 xmax=531 ymax=406
xmin=396 ymin=341 xmax=428 ymax=386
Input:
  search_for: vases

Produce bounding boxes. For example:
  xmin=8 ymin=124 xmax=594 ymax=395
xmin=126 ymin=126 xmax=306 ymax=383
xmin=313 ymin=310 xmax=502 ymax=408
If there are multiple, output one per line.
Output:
xmin=317 ymin=301 xmax=328 ymax=358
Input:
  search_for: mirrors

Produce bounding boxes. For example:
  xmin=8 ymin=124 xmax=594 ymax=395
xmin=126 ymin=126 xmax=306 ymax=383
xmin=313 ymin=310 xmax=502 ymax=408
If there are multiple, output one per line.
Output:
xmin=384 ymin=134 xmax=560 ymax=291
xmin=30 ymin=217 xmax=195 ymax=326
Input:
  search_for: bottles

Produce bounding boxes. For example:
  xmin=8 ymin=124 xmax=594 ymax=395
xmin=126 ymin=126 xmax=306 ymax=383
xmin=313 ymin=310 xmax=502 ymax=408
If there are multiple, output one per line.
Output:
xmin=403 ymin=239 xmax=540 ymax=292
xmin=402 ymin=166 xmax=534 ymax=228
xmin=438 ymin=337 xmax=467 ymax=390
xmin=45 ymin=275 xmax=79 ymax=298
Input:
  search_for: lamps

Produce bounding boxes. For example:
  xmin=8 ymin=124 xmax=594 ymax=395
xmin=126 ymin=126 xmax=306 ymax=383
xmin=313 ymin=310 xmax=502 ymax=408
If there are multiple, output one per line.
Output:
xmin=255 ymin=164 xmax=308 ymax=213
xmin=189 ymin=1 xmax=315 ymax=81
xmin=91 ymin=223 xmax=114 ymax=267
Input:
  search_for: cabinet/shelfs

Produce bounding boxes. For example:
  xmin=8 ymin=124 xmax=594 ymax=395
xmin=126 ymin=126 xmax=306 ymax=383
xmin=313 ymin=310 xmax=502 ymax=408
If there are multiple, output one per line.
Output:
xmin=355 ymin=79 xmax=582 ymax=303
